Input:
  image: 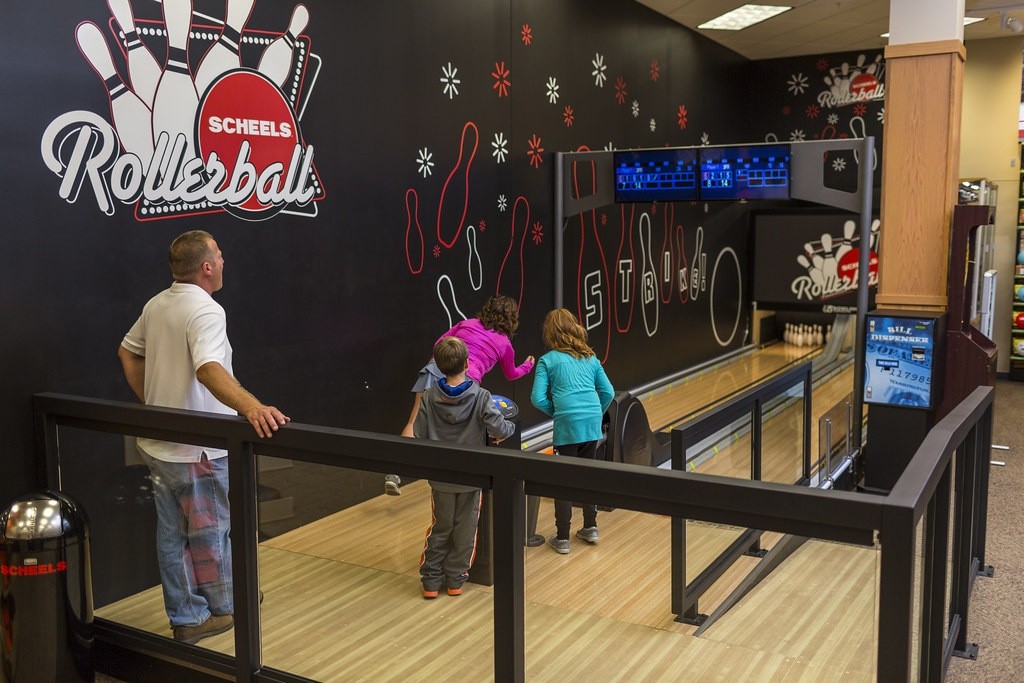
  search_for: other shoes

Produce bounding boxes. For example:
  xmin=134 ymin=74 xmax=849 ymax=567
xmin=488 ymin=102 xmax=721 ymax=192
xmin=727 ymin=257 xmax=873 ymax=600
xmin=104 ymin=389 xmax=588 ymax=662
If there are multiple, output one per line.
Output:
xmin=214 ymin=589 xmax=263 ymax=624
xmin=173 ymin=611 xmax=234 ymax=645
xmin=548 ymin=536 xmax=570 ymax=554
xmin=423 ymin=583 xmax=438 ymax=597
xmin=447 ymin=583 xmax=462 ymax=596
xmin=385 ymin=474 xmax=401 ymax=496
xmin=576 ymin=526 xmax=598 ymax=542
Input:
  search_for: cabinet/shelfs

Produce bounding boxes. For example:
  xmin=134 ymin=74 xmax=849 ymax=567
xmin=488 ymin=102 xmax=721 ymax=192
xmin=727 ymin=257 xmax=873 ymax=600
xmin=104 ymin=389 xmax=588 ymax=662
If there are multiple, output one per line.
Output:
xmin=1009 ymin=141 xmax=1024 ymax=381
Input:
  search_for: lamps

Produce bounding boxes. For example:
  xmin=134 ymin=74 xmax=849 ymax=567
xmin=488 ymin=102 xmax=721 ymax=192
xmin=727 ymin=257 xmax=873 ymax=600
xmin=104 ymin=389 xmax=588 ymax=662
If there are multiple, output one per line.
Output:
xmin=1006 ymin=16 xmax=1022 ymax=33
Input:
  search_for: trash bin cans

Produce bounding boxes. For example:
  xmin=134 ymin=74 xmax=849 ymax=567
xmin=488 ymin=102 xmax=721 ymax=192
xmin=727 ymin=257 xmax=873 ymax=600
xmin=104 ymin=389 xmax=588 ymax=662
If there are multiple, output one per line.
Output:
xmin=1 ymin=489 xmax=95 ymax=682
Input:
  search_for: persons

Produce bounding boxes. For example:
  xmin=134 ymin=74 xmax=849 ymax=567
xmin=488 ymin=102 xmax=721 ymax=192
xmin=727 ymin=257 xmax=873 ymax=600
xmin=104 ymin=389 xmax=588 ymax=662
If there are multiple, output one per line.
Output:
xmin=530 ymin=308 xmax=615 ymax=554
xmin=385 ymin=294 xmax=535 ymax=496
xmin=117 ymin=230 xmax=290 ymax=645
xmin=413 ymin=336 xmax=515 ymax=600
